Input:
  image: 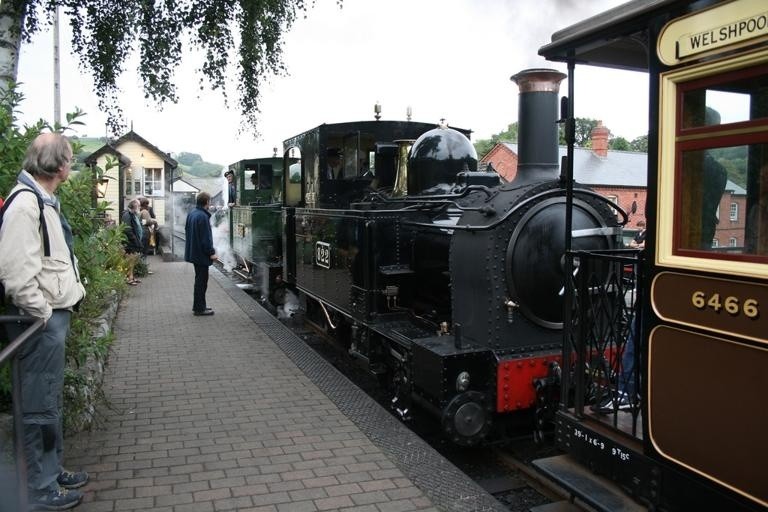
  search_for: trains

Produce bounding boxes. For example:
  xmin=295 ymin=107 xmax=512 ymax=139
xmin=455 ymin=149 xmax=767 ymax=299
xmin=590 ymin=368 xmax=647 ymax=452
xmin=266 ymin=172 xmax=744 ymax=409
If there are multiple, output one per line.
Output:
xmin=213 ymin=0 xmax=768 ymax=511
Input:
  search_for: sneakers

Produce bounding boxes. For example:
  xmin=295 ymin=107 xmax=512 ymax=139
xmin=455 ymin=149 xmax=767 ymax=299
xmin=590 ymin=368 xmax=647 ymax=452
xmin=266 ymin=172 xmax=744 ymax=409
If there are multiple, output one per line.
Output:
xmin=57 ymin=470 xmax=89 ymax=489
xmin=28 ymin=488 xmax=84 ymax=510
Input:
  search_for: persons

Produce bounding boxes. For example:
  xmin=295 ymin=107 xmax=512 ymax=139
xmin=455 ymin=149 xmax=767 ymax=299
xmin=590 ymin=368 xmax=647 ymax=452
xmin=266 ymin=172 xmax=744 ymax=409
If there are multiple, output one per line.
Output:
xmin=117 ymin=195 xmax=159 ymax=287
xmin=592 ymin=103 xmax=728 ymax=415
xmin=337 ymin=150 xmax=374 ymax=180
xmin=0 ymin=131 xmax=93 ymax=512
xmin=327 ymin=148 xmax=345 ymax=181
xmin=250 ymin=172 xmax=257 ymax=189
xmin=223 ymin=170 xmax=236 ymax=208
xmin=182 ymin=191 xmax=217 ymax=315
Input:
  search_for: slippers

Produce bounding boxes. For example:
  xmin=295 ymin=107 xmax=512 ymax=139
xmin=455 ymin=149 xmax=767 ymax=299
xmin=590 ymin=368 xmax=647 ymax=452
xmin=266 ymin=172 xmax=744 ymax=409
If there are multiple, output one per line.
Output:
xmin=127 ymin=278 xmax=141 ymax=285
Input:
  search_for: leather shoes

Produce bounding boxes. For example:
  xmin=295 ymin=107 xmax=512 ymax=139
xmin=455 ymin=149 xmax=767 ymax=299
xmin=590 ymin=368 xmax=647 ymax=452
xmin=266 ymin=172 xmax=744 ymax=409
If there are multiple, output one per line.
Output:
xmin=192 ymin=306 xmax=214 ymax=316
